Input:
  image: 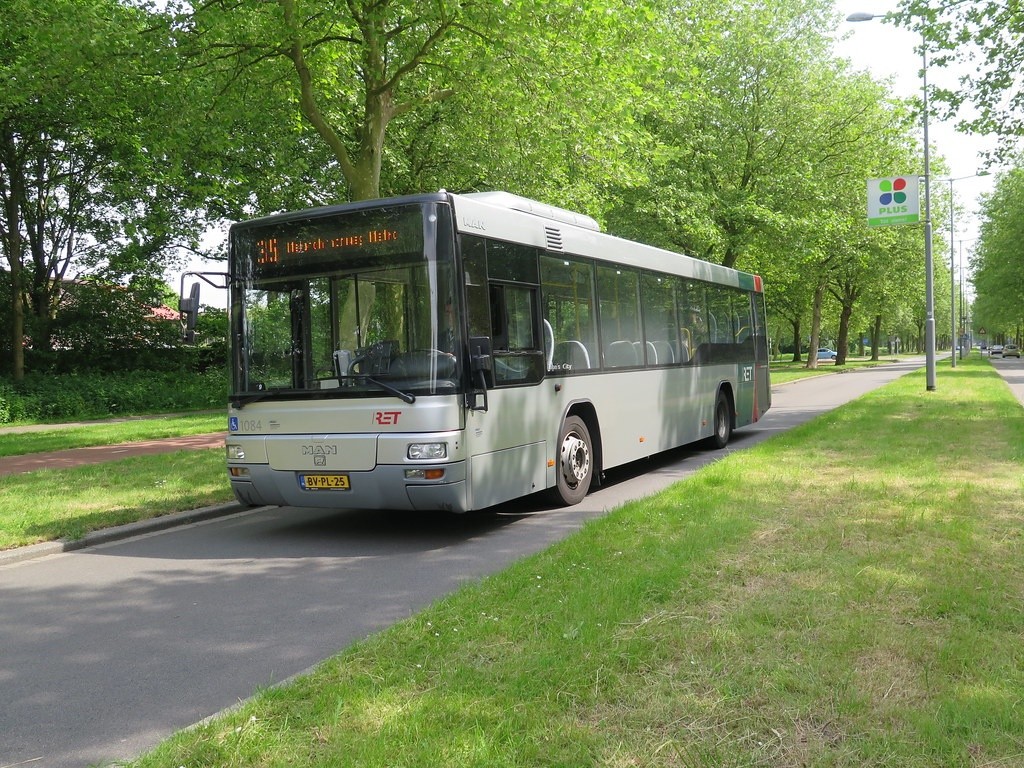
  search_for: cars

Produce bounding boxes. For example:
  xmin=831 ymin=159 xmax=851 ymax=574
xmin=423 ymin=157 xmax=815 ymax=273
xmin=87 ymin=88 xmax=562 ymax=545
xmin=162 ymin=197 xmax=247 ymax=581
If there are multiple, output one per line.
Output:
xmin=977 ymin=341 xmax=1021 ymax=359
xmin=817 ymin=347 xmax=837 ymax=360
xmin=956 ymin=345 xmax=965 ymax=350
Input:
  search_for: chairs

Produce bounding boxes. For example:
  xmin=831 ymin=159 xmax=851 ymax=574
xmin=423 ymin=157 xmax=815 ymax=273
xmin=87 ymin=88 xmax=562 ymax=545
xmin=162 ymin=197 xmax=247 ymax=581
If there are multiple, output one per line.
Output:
xmin=553 ymin=340 xmax=590 ymax=369
xmin=633 ymin=340 xmax=657 ymax=366
xmin=652 ymin=341 xmax=674 ymax=365
xmin=605 ymin=340 xmax=639 ymax=367
xmin=669 ymin=340 xmax=690 ymax=363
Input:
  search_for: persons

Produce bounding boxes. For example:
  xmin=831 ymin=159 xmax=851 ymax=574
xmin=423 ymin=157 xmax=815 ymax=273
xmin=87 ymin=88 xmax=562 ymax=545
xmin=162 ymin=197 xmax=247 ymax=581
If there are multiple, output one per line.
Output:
xmin=437 ymin=298 xmax=457 ymax=356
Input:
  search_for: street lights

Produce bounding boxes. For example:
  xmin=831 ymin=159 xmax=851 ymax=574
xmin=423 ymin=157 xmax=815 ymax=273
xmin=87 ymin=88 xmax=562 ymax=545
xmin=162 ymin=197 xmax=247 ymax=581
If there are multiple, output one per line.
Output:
xmin=844 ymin=9 xmax=939 ymax=394
xmin=916 ymin=169 xmax=993 ymax=366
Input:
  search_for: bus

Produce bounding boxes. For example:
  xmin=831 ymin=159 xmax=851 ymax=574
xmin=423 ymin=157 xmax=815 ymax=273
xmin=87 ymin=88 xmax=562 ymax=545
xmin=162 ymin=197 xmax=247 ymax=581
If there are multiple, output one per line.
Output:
xmin=174 ymin=187 xmax=775 ymax=516
